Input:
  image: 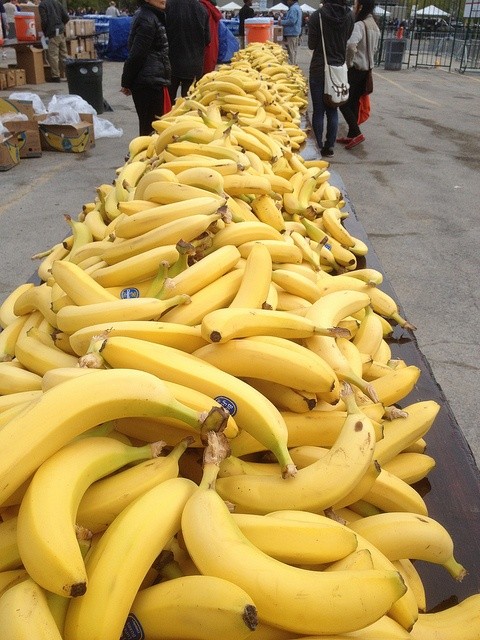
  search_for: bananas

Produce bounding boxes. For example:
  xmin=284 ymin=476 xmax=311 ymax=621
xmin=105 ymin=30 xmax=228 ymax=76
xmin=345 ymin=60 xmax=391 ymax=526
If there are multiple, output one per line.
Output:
xmin=0 ymin=173 xmax=480 ymax=640
xmin=116 ymin=39 xmax=331 ymax=173
xmin=38 ymin=127 xmax=89 ymax=153
xmin=18 ymin=136 xmax=26 ymax=150
xmin=7 ymin=144 xmax=20 ymax=164
xmin=0 ymin=98 xmax=21 ymax=116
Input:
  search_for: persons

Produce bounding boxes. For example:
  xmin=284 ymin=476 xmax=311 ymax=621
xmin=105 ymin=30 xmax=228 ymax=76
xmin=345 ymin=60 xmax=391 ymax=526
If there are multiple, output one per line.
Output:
xmin=0 ymin=1 xmax=9 ymax=30
xmin=12 ymin=0 xmax=22 ymax=12
xmin=119 ymin=1 xmax=174 ymax=136
xmin=376 ymin=14 xmax=409 ymax=38
xmin=278 ymin=0 xmax=303 ymax=64
xmin=263 ymin=10 xmax=283 ymax=25
xmin=38 ymin=0 xmax=70 ymax=82
xmin=34 ymin=0 xmax=42 ymax=6
xmin=200 ymin=0 xmax=224 ymax=74
xmin=23 ymin=0 xmax=35 ymax=6
xmin=4 ymin=0 xmax=17 ymax=38
xmin=335 ymin=0 xmax=382 ymax=149
xmin=166 ymin=0 xmax=211 ymax=106
xmin=106 ymin=0 xmax=128 ymax=17
xmin=211 ymin=0 xmax=240 ymax=63
xmin=308 ymin=0 xmax=356 ymax=156
xmin=68 ymin=5 xmax=99 ymax=16
xmin=121 ymin=6 xmax=129 ymax=17
xmin=239 ymin=0 xmax=259 ymax=49
xmin=425 ymin=15 xmax=434 ymax=40
xmin=226 ymin=10 xmax=234 ymax=19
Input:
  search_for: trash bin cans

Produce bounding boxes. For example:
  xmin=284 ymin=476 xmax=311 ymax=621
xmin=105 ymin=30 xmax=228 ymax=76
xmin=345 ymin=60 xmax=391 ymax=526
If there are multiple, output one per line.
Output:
xmin=385 ymin=36 xmax=406 ymax=72
xmin=65 ymin=58 xmax=103 ymax=115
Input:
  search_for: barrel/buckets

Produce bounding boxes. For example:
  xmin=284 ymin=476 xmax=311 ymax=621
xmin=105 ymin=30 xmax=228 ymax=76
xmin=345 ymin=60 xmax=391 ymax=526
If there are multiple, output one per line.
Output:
xmin=244 ymin=18 xmax=271 ymax=47
xmin=13 ymin=11 xmax=37 ymax=42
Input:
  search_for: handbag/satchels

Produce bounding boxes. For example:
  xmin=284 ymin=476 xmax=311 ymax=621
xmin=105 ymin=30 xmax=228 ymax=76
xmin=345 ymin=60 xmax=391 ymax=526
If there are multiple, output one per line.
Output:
xmin=348 ymin=67 xmax=374 ymax=95
xmin=322 ymin=62 xmax=351 ymax=108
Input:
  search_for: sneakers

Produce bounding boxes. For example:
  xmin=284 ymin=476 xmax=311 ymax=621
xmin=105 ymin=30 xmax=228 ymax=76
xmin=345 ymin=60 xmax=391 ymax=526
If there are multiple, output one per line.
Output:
xmin=322 ymin=146 xmax=335 ymax=157
xmin=342 ymin=133 xmax=365 ymax=149
xmin=336 ymin=138 xmax=352 ymax=143
